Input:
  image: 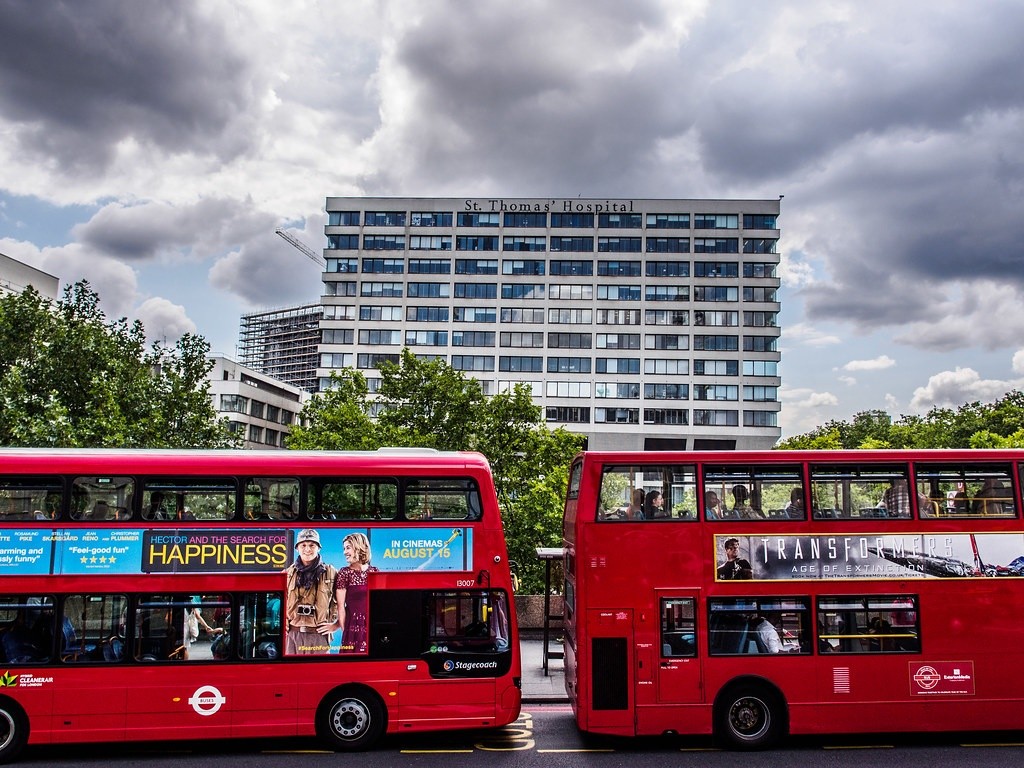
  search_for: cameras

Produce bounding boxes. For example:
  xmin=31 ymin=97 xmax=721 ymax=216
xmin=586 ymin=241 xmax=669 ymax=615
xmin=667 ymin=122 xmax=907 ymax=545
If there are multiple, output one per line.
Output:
xmin=298 ymin=605 xmax=315 ymax=617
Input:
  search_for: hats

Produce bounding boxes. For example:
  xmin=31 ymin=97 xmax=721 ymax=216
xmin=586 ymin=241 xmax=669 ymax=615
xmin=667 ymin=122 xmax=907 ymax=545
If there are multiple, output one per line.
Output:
xmin=294 ymin=529 xmax=322 ymax=550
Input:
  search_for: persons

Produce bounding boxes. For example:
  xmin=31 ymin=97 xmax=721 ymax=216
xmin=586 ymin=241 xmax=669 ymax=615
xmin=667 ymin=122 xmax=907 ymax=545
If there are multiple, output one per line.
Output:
xmin=892 ymin=596 xmax=917 ymax=625
xmin=817 ymin=619 xmax=845 ymax=652
xmin=929 ymin=490 xmax=946 ymax=514
xmin=279 ymin=529 xmax=342 ymax=655
xmin=706 ymin=491 xmax=722 ymax=520
xmin=881 ymin=477 xmax=928 ymax=518
xmin=785 ymin=487 xmax=805 ymax=518
xmin=624 ymin=487 xmax=669 ymax=519
xmin=86 ymin=500 xmax=109 ymax=520
xmin=731 ymin=485 xmax=764 ymax=519
xmin=166 ymin=594 xmax=281 ymax=658
xmin=716 ymin=537 xmax=754 ymax=580
xmin=143 ymin=492 xmax=171 ymax=520
xmin=281 ymin=495 xmax=299 ymax=519
xmin=461 ymin=591 xmax=509 ymax=637
xmin=755 ymin=610 xmax=802 ymax=653
xmin=952 ymin=476 xmax=1015 ymax=515
xmin=859 ymin=616 xmax=901 ymax=650
xmin=334 ymin=533 xmax=380 ymax=653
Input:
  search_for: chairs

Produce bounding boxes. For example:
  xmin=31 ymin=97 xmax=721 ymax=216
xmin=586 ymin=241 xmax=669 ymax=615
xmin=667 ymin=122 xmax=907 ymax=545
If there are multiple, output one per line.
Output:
xmin=681 ymin=611 xmax=773 ymax=654
xmin=633 ymin=503 xmax=890 ymax=521
xmin=0 ymin=615 xmax=169 ymax=662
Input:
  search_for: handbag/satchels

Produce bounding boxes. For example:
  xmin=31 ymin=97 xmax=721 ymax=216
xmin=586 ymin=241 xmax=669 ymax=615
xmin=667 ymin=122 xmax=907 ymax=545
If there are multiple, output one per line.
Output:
xmin=188 ymin=608 xmax=200 ymax=637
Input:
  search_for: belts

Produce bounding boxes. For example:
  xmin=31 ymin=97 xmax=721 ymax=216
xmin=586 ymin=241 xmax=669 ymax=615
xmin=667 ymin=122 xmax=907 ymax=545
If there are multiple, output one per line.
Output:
xmin=289 ymin=624 xmax=321 ymax=634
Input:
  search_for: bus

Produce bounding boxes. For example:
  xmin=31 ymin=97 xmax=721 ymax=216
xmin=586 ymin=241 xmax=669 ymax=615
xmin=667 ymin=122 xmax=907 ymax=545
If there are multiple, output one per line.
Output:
xmin=0 ymin=436 xmax=524 ymax=766
xmin=561 ymin=444 xmax=1024 ymax=752
